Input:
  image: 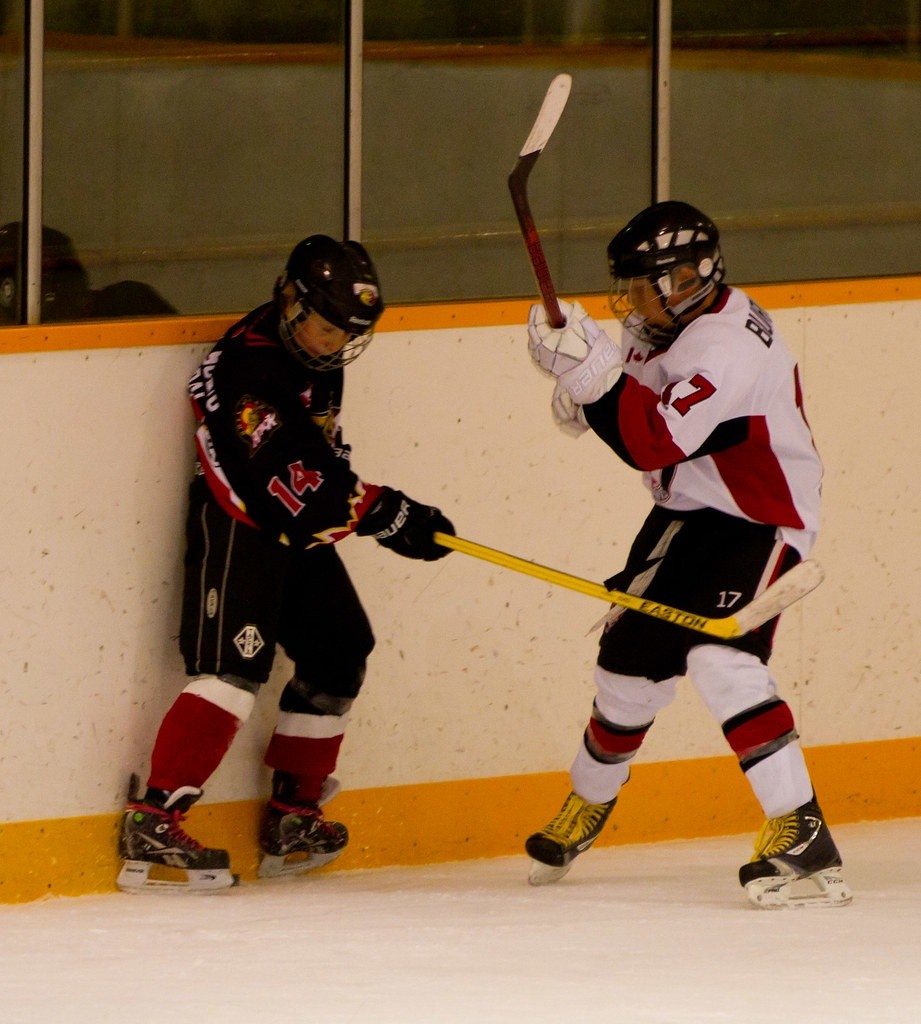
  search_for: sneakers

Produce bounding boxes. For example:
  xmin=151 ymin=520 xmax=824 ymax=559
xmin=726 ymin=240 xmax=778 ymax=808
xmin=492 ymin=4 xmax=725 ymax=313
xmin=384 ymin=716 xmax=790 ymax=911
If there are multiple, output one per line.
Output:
xmin=258 ymin=776 xmax=348 ymax=877
xmin=738 ymin=783 xmax=852 ymax=909
xmin=115 ymin=772 xmax=234 ymax=894
xmin=524 ymin=771 xmax=632 ymax=885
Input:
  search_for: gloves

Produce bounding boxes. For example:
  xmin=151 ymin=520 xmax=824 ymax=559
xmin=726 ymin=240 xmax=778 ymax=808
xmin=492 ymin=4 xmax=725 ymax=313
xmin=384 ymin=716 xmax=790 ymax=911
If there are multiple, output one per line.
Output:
xmin=329 ymin=442 xmax=353 ymax=470
xmin=353 ymin=482 xmax=456 ymax=562
xmin=551 ymin=376 xmax=591 ymax=439
xmin=525 ymin=294 xmax=625 ymax=404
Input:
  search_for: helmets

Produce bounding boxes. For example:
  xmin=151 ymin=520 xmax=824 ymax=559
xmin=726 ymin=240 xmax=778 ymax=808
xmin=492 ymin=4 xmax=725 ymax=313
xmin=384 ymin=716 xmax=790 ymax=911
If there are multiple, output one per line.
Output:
xmin=607 ymin=200 xmax=726 ymax=351
xmin=272 ymin=233 xmax=386 ymax=371
xmin=0 ymin=221 xmax=87 ymax=327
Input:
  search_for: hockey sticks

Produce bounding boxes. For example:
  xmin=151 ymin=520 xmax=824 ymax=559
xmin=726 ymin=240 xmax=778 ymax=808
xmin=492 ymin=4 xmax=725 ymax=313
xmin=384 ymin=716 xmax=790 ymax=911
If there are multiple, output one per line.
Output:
xmin=430 ymin=527 xmax=827 ymax=634
xmin=503 ymin=73 xmax=572 ymax=328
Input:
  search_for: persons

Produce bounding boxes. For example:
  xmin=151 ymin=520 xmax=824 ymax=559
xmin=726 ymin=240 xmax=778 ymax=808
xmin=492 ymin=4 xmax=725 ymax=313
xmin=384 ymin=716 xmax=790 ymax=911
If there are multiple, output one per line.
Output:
xmin=518 ymin=201 xmax=856 ymax=913
xmin=0 ymin=220 xmax=180 ymax=325
xmin=115 ymin=233 xmax=462 ymax=897
